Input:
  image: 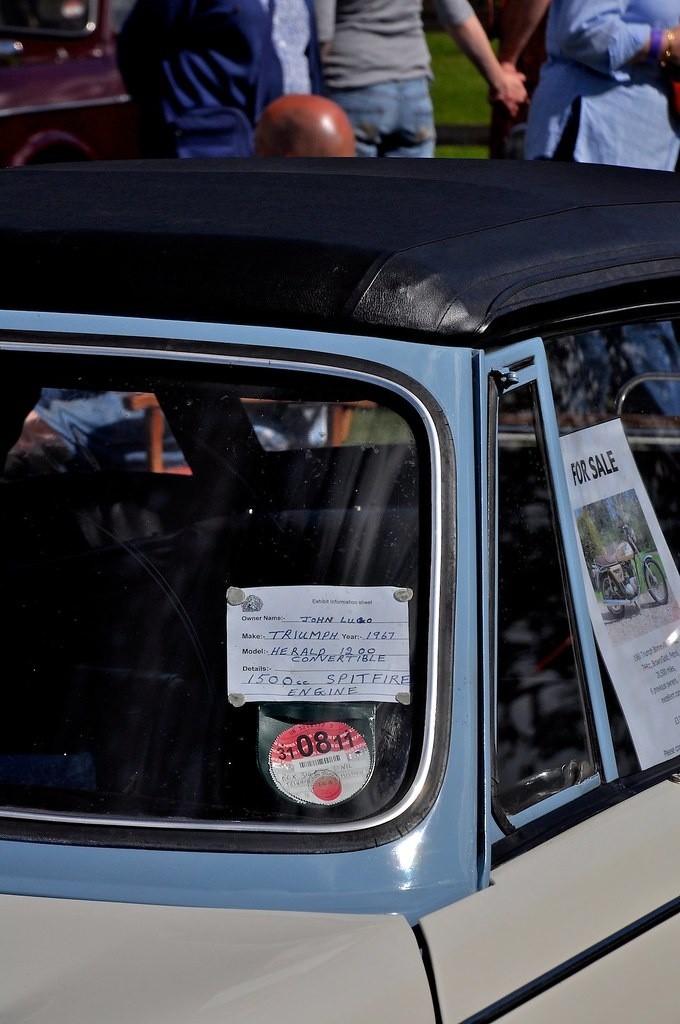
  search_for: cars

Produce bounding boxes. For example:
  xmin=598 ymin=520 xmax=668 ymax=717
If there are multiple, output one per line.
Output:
xmin=0 ymin=152 xmax=680 ymax=1024
xmin=0 ymin=0 xmax=172 ymax=165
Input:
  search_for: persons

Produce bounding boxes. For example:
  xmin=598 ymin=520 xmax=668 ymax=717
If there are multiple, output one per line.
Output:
xmin=9 ymin=93 xmax=357 ymax=466
xmin=114 ymin=0 xmax=323 ymax=156
xmin=489 ymin=0 xmax=549 ymax=161
xmin=313 ymin=0 xmax=529 ymax=163
xmin=521 ymin=0 xmax=680 ymax=420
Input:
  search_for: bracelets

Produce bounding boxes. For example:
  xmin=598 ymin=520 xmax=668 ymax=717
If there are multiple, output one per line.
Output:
xmin=659 ymin=28 xmax=674 ymax=67
xmin=648 ymin=29 xmax=663 ymax=64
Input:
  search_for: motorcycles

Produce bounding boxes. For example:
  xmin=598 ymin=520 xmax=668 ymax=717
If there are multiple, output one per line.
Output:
xmin=591 ymin=520 xmax=669 ymax=618
